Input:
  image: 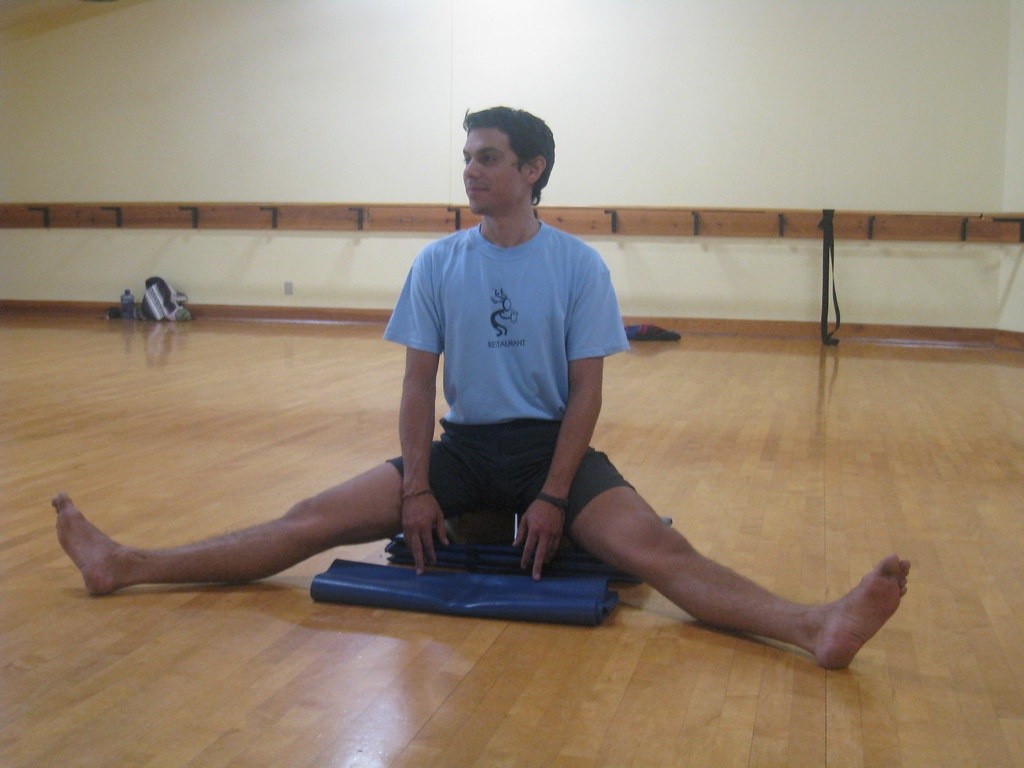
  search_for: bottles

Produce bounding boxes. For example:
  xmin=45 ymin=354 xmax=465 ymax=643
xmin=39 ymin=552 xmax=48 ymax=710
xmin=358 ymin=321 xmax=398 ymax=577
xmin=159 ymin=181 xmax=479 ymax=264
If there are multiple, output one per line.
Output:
xmin=120 ymin=290 xmax=135 ymax=318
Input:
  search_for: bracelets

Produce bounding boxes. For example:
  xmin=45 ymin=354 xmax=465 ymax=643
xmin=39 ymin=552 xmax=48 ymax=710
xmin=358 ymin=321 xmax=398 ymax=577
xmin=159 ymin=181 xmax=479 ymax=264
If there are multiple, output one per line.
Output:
xmin=536 ymin=491 xmax=567 ymax=509
xmin=402 ymin=488 xmax=431 ymax=498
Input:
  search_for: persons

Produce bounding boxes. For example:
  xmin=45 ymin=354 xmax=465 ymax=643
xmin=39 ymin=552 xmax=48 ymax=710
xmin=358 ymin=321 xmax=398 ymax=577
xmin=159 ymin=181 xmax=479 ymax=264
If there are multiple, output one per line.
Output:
xmin=51 ymin=108 xmax=911 ymax=669
